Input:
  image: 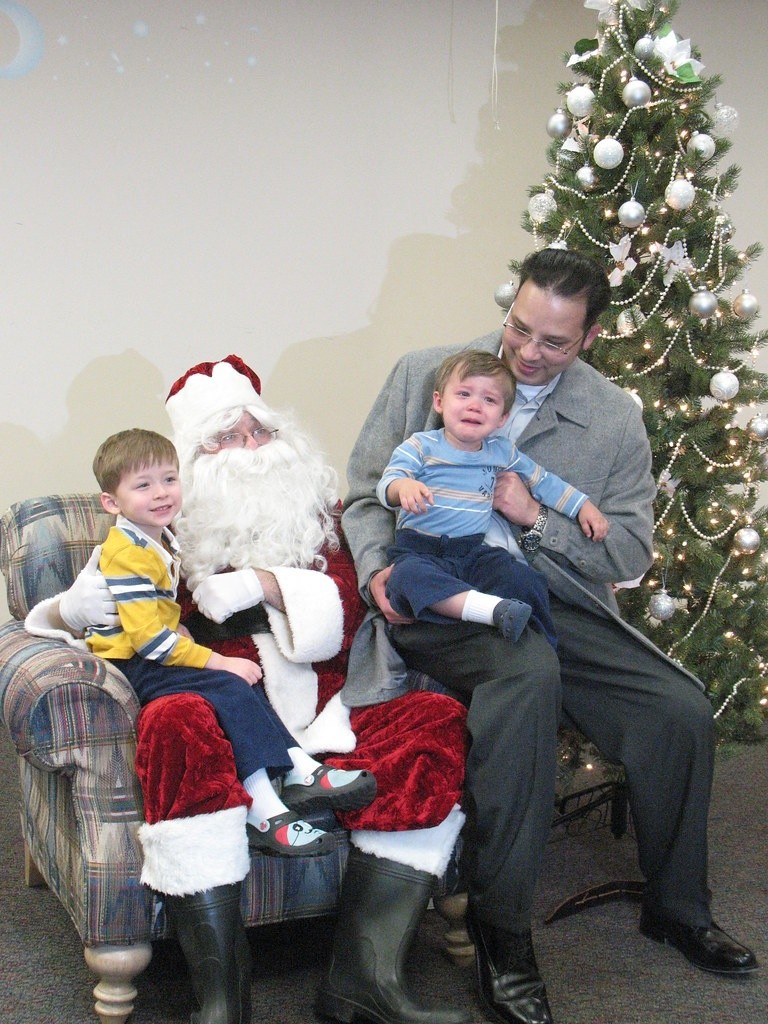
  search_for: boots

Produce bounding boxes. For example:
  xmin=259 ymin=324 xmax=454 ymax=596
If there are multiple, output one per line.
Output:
xmin=312 ymin=810 xmax=473 ymax=1024
xmin=138 ymin=805 xmax=253 ymax=1024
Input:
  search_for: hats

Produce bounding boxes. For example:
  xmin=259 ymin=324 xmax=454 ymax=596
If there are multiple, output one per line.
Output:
xmin=165 ymin=355 xmax=268 ymax=437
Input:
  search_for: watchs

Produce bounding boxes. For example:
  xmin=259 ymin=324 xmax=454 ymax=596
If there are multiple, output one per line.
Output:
xmin=519 ymin=505 xmax=548 ymax=551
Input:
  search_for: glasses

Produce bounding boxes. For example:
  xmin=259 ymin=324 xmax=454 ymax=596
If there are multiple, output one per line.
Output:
xmin=218 ymin=427 xmax=280 ymax=450
xmin=502 ymin=299 xmax=592 ymax=358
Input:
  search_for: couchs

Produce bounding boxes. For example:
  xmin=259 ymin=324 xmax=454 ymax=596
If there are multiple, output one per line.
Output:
xmin=0 ymin=487 xmax=477 ymax=1023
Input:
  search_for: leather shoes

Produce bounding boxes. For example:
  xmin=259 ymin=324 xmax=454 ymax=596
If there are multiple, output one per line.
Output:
xmin=464 ymin=898 xmax=553 ymax=1024
xmin=639 ymin=903 xmax=761 ymax=974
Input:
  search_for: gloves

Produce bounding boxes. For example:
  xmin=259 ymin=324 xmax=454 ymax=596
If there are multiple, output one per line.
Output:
xmin=59 ymin=545 xmax=122 ymax=631
xmin=191 ymin=567 xmax=266 ymax=625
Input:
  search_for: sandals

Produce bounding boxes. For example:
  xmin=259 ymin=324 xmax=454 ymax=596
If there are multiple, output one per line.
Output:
xmin=280 ymin=764 xmax=377 ymax=815
xmin=245 ymin=808 xmax=335 ymax=857
xmin=494 ymin=596 xmax=531 ymax=642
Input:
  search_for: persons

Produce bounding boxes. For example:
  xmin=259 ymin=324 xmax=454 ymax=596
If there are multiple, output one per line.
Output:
xmin=341 ymin=248 xmax=759 ymax=1024
xmin=23 ymin=354 xmax=470 ymax=1024
xmin=83 ymin=428 xmax=377 ymax=858
xmin=375 ymin=352 xmax=609 ymax=652
